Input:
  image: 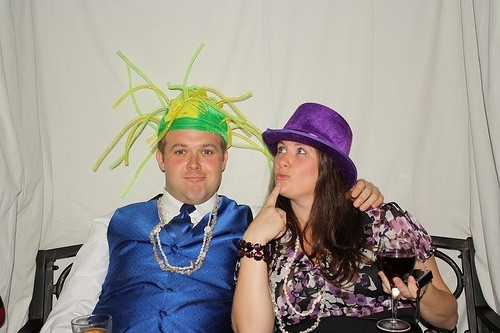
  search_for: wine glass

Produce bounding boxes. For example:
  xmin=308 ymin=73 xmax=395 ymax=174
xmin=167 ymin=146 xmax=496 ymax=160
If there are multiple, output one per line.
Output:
xmin=377 ymin=236 xmax=417 ymax=333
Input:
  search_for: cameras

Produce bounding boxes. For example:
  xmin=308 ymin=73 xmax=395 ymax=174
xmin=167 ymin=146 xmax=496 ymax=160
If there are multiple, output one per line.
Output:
xmin=404 ymin=267 xmax=432 ymax=290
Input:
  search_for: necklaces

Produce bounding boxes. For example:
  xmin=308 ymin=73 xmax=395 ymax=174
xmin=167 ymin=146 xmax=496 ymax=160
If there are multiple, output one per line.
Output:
xmin=150 ymin=193 xmax=220 ymax=275
xmin=271 ymin=231 xmax=329 ymax=333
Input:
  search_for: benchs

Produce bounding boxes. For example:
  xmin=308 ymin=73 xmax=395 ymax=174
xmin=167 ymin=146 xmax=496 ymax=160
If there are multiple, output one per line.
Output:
xmin=17 ymin=236 xmax=500 ymax=333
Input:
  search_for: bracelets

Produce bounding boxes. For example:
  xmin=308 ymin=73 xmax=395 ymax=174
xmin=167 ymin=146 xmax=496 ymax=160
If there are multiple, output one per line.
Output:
xmin=238 ymin=241 xmax=269 ymax=263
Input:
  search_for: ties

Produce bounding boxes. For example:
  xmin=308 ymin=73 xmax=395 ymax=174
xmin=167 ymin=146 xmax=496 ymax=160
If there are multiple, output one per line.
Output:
xmin=164 ymin=203 xmax=196 ymax=243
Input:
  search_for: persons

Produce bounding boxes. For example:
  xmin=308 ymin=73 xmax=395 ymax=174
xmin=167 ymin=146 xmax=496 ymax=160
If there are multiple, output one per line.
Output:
xmin=231 ymin=102 xmax=459 ymax=333
xmin=40 ymin=100 xmax=384 ymax=333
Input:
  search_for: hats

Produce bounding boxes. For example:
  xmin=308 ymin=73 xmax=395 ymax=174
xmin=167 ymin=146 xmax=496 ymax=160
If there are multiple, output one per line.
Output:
xmin=262 ymin=102 xmax=357 ymax=188
xmin=93 ymin=42 xmax=275 ymax=200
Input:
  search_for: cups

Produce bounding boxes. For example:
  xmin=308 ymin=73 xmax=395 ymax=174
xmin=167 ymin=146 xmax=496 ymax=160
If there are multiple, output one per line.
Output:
xmin=71 ymin=313 xmax=113 ymax=333
